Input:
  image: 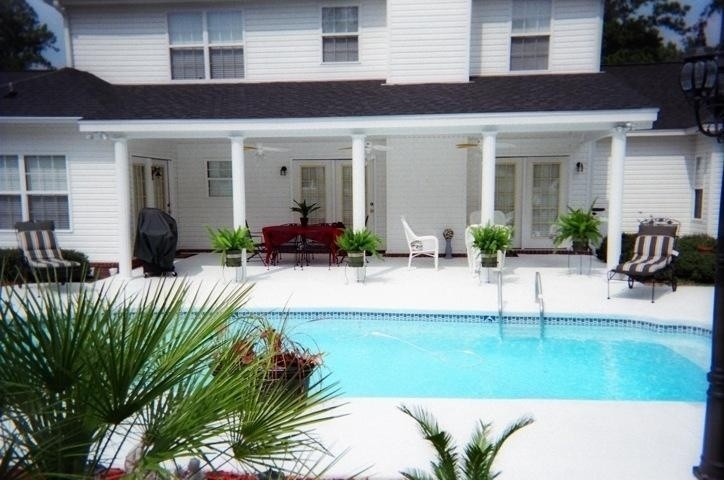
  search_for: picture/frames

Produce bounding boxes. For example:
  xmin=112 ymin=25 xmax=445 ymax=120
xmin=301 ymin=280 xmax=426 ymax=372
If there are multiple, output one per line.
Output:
xmin=261 ymin=222 xmax=342 ymax=269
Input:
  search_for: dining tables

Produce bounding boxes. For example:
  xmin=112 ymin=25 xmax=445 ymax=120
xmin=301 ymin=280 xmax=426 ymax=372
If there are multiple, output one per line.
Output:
xmin=205 ymin=222 xmax=257 ymax=270
xmin=286 ymin=195 xmax=322 ymax=225
xmin=468 ymin=219 xmax=514 ymax=270
xmin=547 ymin=195 xmax=604 ymax=257
xmin=333 ymin=224 xmax=385 ymax=267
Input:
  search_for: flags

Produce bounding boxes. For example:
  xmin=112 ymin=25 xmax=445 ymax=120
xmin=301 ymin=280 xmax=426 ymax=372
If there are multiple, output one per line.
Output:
xmin=208 ymin=358 xmax=312 ymax=408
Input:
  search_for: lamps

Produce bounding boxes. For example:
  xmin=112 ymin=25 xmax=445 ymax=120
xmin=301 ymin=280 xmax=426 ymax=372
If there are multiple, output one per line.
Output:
xmin=245 ymin=219 xmax=281 ymax=268
xmin=606 ymin=216 xmax=684 ymax=303
xmin=399 ymin=213 xmax=441 ymax=274
xmin=13 ymin=218 xmax=88 ymax=294
